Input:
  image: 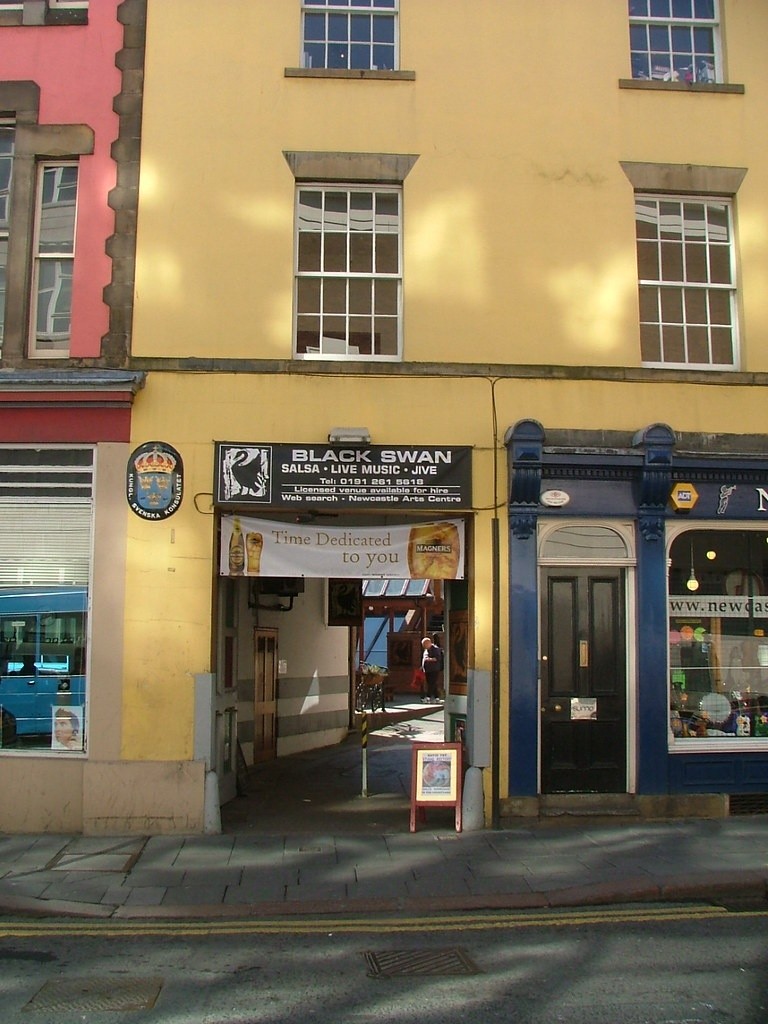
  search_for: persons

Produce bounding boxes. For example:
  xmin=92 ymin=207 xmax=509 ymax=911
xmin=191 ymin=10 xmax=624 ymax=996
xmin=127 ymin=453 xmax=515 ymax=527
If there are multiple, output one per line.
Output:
xmin=420 ymin=637 xmax=444 ymax=704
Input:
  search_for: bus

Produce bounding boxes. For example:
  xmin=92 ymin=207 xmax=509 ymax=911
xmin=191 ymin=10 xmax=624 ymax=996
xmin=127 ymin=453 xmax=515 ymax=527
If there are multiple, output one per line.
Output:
xmin=0 ymin=586 xmax=90 ymax=746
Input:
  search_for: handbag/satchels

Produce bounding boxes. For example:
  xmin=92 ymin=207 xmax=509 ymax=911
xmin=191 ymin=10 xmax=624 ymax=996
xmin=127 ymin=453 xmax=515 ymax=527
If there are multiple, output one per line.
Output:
xmin=411 ymin=666 xmax=426 ymax=689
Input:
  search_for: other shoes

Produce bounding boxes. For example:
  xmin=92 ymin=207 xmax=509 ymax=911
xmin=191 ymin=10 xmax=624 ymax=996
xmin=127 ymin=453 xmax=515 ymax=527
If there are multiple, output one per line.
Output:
xmin=431 ymin=698 xmax=440 ymax=703
xmin=420 ymin=697 xmax=430 ymax=703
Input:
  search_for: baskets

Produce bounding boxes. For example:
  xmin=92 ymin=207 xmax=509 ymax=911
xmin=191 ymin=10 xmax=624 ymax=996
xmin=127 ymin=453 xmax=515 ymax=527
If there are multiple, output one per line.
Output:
xmin=362 ymin=667 xmax=385 ymax=686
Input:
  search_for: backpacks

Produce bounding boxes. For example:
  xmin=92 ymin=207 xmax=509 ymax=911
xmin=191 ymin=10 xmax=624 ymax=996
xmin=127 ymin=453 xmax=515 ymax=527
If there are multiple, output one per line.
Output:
xmin=431 ymin=646 xmax=445 ymax=671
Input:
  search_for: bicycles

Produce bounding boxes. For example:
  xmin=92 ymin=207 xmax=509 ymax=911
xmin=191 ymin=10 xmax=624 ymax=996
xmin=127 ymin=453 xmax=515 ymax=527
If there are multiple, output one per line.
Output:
xmin=356 ymin=661 xmax=390 ymax=713
